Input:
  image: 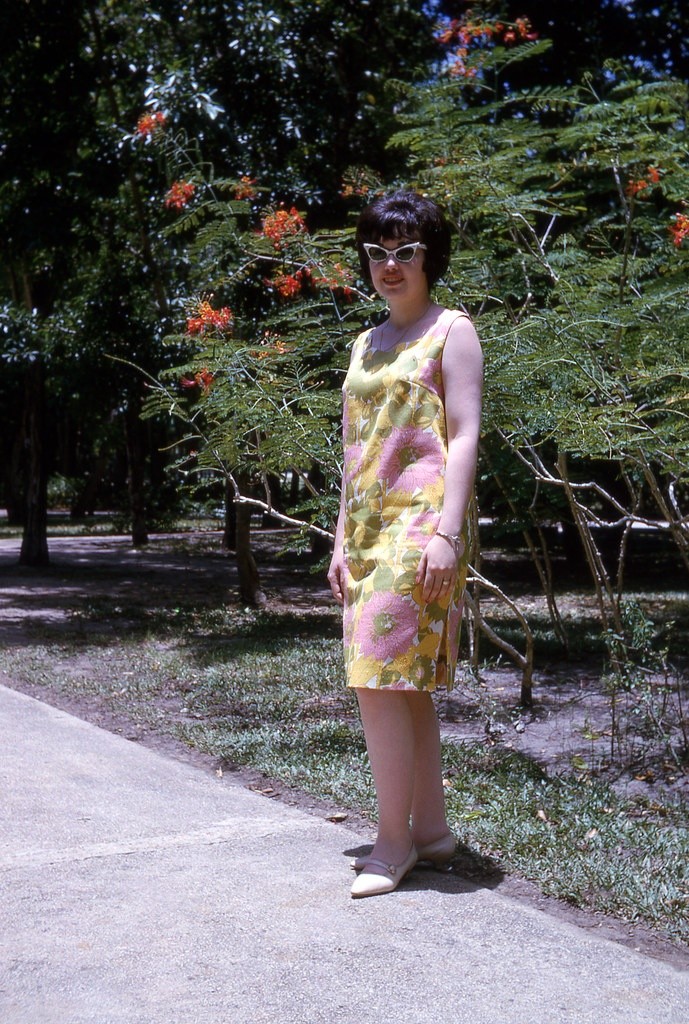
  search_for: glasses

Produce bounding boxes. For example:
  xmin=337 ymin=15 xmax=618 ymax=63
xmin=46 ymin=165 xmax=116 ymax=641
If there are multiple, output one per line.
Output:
xmin=364 ymin=242 xmax=428 ymax=262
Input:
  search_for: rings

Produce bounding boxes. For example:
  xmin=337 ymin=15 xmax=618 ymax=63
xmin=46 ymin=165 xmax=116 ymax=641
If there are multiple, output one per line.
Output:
xmin=442 ymin=580 xmax=450 ymax=585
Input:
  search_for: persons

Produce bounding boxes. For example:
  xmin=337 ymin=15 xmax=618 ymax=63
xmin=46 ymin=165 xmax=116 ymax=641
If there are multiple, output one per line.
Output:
xmin=324 ymin=186 xmax=486 ymax=900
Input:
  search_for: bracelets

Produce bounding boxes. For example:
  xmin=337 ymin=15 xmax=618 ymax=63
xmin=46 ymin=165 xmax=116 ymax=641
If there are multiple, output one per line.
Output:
xmin=434 ymin=531 xmax=462 ymax=545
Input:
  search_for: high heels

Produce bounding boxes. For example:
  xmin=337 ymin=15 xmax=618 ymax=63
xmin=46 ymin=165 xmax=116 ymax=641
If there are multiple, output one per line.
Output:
xmin=351 ymin=841 xmax=419 ymax=897
xmin=351 ymin=834 xmax=459 ymax=871
